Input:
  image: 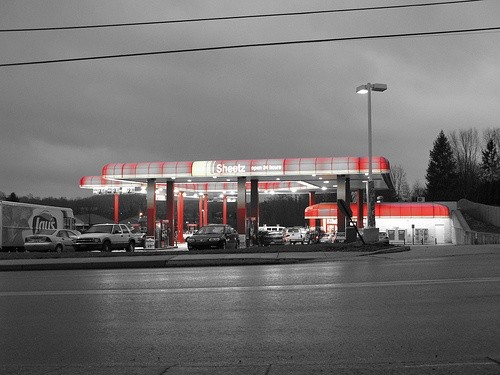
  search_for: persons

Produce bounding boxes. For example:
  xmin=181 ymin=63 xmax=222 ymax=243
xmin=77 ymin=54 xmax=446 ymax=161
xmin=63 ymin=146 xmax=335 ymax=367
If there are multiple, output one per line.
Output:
xmin=258 ymin=231 xmax=273 ymax=246
xmin=284 ymin=235 xmax=290 ymax=242
xmin=283 ymin=226 xmax=288 ymax=237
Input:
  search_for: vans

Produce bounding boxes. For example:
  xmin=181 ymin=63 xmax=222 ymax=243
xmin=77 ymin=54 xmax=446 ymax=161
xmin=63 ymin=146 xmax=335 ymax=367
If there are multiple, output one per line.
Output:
xmin=259 ymin=226 xmax=346 ymax=243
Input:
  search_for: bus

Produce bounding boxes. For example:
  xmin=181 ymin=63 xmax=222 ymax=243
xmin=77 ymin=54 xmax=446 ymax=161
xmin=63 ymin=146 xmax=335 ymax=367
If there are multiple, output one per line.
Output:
xmin=0 ymin=201 xmax=76 ymax=249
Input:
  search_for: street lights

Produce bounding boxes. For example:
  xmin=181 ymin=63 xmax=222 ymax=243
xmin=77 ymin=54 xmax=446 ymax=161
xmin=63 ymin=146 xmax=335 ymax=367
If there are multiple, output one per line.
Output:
xmin=356 ymin=82 xmax=388 ymax=226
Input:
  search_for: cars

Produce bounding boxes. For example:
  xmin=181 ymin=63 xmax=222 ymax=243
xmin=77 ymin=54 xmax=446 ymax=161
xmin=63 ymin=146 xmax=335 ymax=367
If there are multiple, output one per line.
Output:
xmin=24 ymin=229 xmax=78 ymax=252
xmin=378 ymin=232 xmax=390 ymax=243
xmin=183 ymin=224 xmax=239 ymax=250
xmin=73 ymin=224 xmax=146 ymax=254
